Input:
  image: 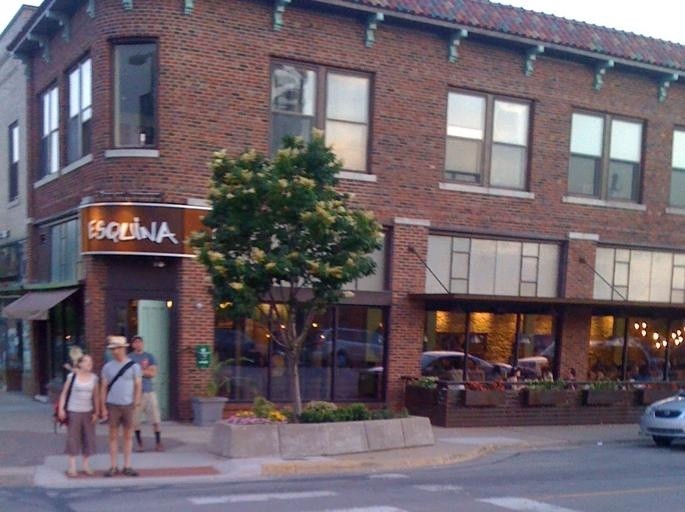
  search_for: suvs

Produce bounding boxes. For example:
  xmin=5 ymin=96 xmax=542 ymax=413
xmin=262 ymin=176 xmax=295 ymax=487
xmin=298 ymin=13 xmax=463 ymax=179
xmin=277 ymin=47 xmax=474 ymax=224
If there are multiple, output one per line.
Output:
xmin=518 ymin=337 xmax=671 ymax=382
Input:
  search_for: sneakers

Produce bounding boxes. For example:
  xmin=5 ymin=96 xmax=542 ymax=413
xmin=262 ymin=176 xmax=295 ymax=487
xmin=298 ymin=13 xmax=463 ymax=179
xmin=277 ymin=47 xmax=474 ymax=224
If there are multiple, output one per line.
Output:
xmin=154 ymin=444 xmax=164 ymax=451
xmin=136 ymin=444 xmax=145 ymax=452
xmin=66 ymin=467 xmax=138 ymax=478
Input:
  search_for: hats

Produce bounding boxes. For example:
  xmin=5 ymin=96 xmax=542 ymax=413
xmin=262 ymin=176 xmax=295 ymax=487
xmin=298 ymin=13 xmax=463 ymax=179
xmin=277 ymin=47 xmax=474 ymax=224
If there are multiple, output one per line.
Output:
xmin=106 ymin=336 xmax=130 ymax=349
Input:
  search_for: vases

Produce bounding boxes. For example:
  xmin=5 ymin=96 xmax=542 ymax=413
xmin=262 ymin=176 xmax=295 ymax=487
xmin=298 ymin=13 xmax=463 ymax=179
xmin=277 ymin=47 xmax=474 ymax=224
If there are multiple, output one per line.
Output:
xmin=464 ymin=390 xmax=504 ymax=406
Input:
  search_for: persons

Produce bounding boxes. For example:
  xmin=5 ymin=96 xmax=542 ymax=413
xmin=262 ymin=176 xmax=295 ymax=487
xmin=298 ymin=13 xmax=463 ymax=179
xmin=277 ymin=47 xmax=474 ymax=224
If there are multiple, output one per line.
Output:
xmin=425 ymin=356 xmax=676 ymax=392
xmin=58 ymin=334 xmax=164 ymax=477
xmin=244 ymin=341 xmax=316 ymax=403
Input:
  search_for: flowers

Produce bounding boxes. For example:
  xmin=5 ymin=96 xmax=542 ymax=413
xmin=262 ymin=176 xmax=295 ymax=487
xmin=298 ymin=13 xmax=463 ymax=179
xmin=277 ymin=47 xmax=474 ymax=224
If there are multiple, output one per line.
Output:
xmin=464 ymin=378 xmax=506 ymax=391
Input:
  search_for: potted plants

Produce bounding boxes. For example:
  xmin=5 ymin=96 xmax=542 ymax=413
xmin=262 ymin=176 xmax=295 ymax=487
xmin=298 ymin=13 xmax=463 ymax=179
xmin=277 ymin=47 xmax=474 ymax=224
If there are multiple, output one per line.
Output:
xmin=190 ymin=351 xmax=256 ymax=427
xmin=523 ymin=378 xmax=566 ymax=406
xmin=585 ymin=376 xmax=624 ymax=406
xmin=405 ymin=376 xmax=440 ymax=405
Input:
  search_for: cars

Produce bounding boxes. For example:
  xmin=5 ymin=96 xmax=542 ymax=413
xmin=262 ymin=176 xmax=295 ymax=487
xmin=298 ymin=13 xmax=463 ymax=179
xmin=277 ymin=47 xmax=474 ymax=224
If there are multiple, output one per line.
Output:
xmin=213 ymin=329 xmax=287 ymax=376
xmin=640 ymin=390 xmax=685 ymax=447
xmin=358 ymin=349 xmax=539 ymax=403
xmin=309 ymin=328 xmax=386 ymax=368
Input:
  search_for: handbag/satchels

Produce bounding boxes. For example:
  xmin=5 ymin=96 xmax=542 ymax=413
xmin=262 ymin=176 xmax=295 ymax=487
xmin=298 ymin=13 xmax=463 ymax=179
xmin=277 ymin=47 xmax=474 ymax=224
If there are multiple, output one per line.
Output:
xmin=98 ymin=413 xmax=110 ymax=425
xmin=53 ymin=397 xmax=70 ymax=426
